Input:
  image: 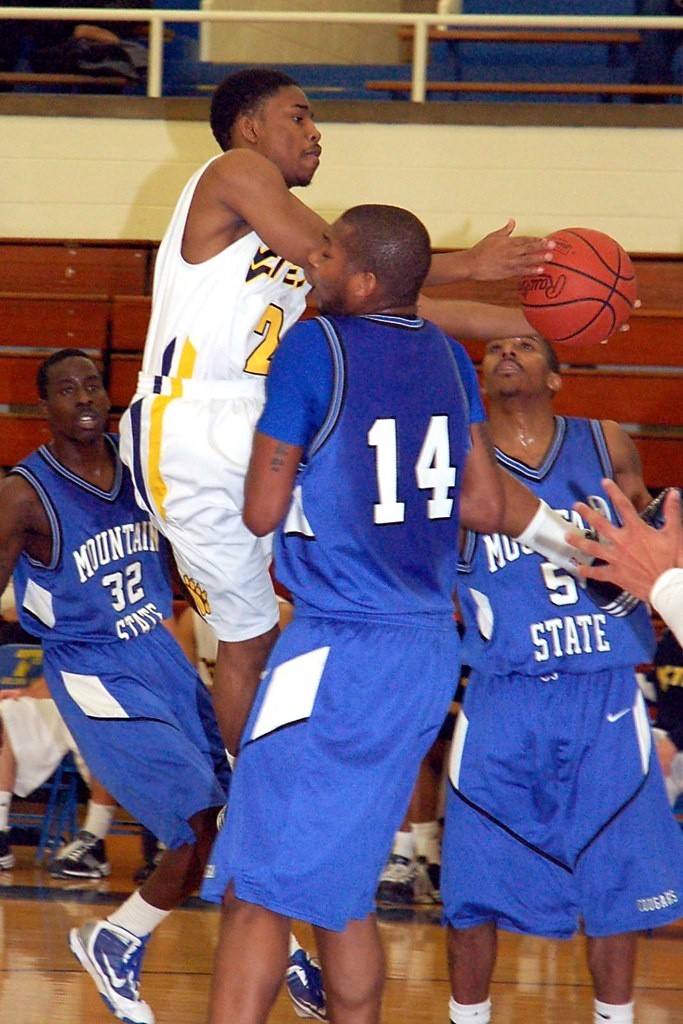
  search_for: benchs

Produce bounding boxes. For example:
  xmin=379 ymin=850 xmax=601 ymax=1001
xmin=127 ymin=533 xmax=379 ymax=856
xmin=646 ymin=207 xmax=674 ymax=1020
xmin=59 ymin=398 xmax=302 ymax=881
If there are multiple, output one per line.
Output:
xmin=0 ymin=244 xmax=683 ymax=504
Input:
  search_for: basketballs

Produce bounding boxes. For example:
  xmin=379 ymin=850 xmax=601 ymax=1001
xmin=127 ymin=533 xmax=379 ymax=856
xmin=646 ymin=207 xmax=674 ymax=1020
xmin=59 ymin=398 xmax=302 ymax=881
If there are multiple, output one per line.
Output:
xmin=515 ymin=227 xmax=639 ymax=349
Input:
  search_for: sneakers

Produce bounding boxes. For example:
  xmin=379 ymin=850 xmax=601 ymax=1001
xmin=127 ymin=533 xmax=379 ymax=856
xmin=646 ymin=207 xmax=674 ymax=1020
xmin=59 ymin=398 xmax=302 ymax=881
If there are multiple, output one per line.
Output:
xmin=134 ymin=841 xmax=167 ymax=883
xmin=376 ymin=849 xmax=444 ymax=903
xmin=284 ymin=932 xmax=328 ymax=1024
xmin=44 ymin=830 xmax=112 ymax=879
xmin=0 ymin=832 xmax=16 ymax=871
xmin=68 ymin=915 xmax=157 ymax=1024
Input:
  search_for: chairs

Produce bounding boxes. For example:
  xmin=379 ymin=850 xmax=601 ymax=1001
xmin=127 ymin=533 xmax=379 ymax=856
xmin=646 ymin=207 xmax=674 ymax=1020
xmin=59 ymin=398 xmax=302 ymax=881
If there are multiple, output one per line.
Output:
xmin=0 ymin=752 xmax=158 ymax=867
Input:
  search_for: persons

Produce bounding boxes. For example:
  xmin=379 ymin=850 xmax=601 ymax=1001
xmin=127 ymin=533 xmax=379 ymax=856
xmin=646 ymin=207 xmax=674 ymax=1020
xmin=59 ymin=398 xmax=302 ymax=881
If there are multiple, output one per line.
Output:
xmin=564 ymin=479 xmax=683 ymax=650
xmin=0 ymin=347 xmax=232 ymax=1024
xmin=442 ymin=333 xmax=683 ymax=1023
xmin=198 ymin=203 xmax=504 ymax=1024
xmin=0 ymin=873 xmax=683 ymax=1024
xmin=110 ymin=65 xmax=559 ymax=748
xmin=0 ymin=578 xmax=682 ymax=905
